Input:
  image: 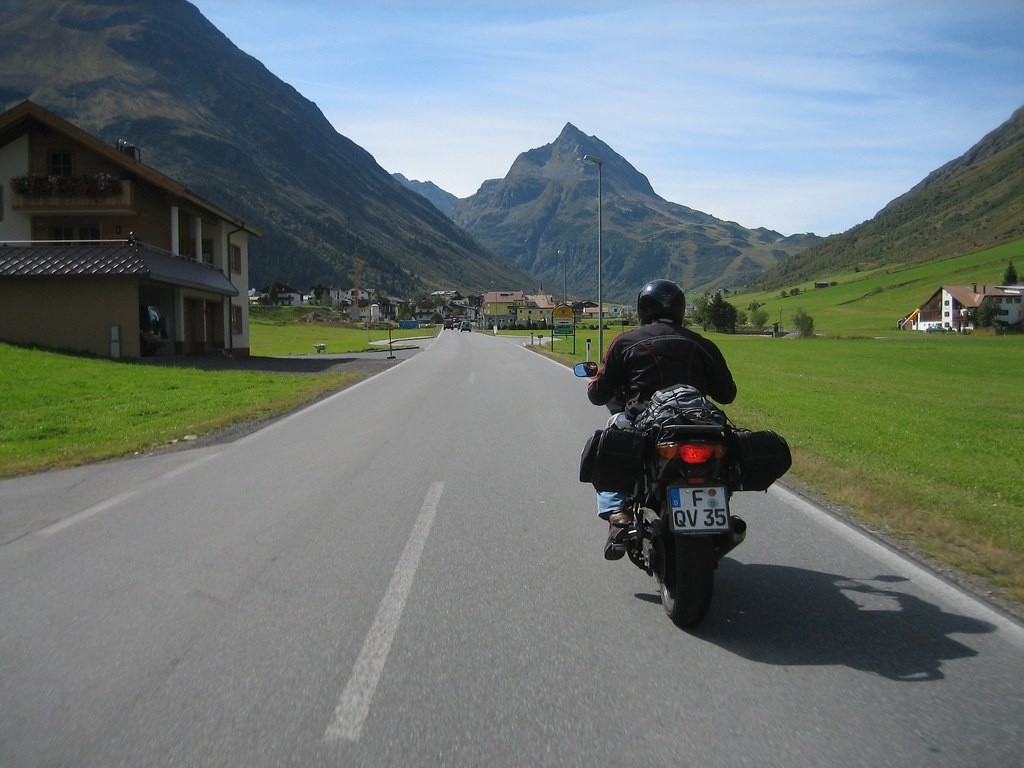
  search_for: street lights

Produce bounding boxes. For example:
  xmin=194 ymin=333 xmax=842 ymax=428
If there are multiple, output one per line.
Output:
xmin=779 ymin=309 xmax=782 ymax=336
xmin=556 ymin=250 xmax=568 ymax=340
xmin=583 ymin=155 xmax=603 ymax=364
xmin=491 ymin=281 xmax=497 ymax=335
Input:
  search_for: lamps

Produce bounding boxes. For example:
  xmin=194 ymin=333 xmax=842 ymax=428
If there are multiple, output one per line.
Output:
xmin=115 ymin=223 xmax=121 ymax=234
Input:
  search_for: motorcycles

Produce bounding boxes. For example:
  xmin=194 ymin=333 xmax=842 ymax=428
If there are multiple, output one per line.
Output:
xmin=573 ymin=361 xmax=747 ymax=621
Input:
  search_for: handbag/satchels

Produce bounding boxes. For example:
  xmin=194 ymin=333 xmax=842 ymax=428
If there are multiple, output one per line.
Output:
xmin=729 ymin=427 xmax=792 ymax=492
xmin=578 ymin=425 xmax=650 ymax=492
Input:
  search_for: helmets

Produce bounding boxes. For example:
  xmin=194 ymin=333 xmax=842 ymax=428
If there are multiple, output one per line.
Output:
xmin=638 ymin=279 xmax=687 ymax=325
xmin=583 ymin=363 xmax=597 ymax=375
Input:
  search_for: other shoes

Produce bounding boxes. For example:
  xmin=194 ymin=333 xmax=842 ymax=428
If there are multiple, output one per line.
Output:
xmin=604 ymin=511 xmax=631 ymax=561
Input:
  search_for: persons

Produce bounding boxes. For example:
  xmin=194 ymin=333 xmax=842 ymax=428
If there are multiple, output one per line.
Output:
xmin=591 ymin=279 xmax=737 ymax=560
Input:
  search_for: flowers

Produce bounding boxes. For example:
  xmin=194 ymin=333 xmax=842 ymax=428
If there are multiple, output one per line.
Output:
xmin=9 ymin=171 xmax=122 ymax=201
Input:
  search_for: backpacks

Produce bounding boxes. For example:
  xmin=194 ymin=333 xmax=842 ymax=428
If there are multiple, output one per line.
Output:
xmin=635 ymin=383 xmax=726 ymax=432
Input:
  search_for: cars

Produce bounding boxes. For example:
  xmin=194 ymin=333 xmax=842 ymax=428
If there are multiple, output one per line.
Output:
xmin=419 ymin=321 xmax=436 ymax=328
xmin=454 ymin=320 xmax=471 ymax=332
xmin=926 ymin=325 xmax=947 ymax=334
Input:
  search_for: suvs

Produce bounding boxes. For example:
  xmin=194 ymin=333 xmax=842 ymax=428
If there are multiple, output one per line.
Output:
xmin=444 ymin=318 xmax=454 ymax=330
xmin=139 ymin=304 xmax=168 ymax=357
